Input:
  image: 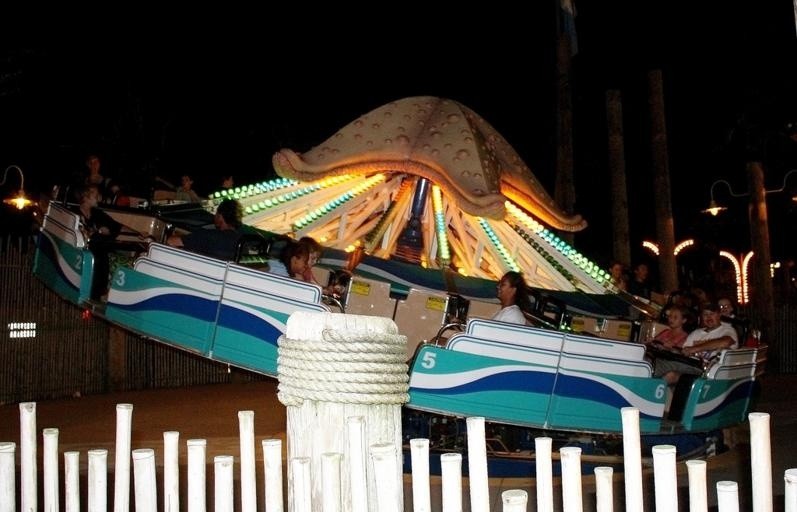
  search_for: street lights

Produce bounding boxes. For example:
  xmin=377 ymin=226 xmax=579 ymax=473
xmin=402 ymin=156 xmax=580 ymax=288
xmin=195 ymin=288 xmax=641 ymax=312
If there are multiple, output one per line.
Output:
xmin=702 ymin=162 xmax=796 ymax=377
xmin=0 ymin=165 xmax=33 ymax=211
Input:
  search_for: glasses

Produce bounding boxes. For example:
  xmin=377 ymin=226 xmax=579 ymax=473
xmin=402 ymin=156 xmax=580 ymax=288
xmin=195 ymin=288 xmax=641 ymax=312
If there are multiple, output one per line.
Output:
xmin=720 ymin=305 xmax=729 ymax=309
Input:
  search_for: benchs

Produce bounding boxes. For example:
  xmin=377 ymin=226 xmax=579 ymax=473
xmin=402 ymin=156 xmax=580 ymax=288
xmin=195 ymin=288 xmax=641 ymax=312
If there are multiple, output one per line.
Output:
xmin=33 ymin=190 xmax=768 ymax=434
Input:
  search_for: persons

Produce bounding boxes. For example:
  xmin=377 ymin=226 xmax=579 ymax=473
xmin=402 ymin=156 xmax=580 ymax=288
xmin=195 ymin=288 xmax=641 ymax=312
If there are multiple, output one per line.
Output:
xmin=256 ymin=235 xmax=345 ymax=313
xmin=219 ymin=174 xmax=233 ymax=190
xmin=86 ymin=154 xmax=118 ymax=196
xmin=609 ymin=258 xmax=747 ymax=420
xmin=177 ymin=175 xmax=201 ymax=204
xmin=167 ymin=200 xmax=250 ymax=264
xmin=405 ymin=271 xmax=532 ymax=367
xmin=69 ymin=184 xmax=144 ymax=314
